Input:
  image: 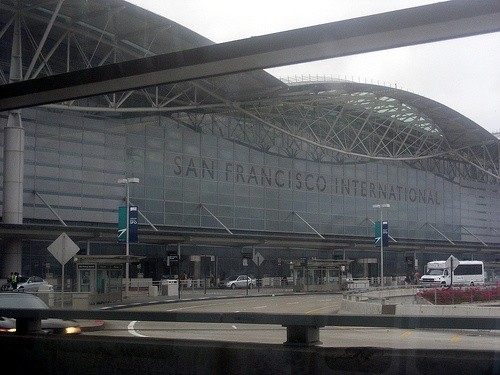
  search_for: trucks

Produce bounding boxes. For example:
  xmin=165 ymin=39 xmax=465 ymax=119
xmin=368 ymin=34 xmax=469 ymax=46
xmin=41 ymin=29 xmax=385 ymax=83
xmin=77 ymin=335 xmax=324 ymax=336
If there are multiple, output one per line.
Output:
xmin=419 ymin=261 xmax=484 ymax=287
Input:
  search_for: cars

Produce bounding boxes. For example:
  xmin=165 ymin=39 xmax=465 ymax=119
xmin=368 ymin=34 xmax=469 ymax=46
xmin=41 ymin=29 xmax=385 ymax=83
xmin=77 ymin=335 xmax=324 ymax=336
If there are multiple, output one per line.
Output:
xmin=0 ymin=293 xmax=81 ymax=334
xmin=2 ymin=276 xmax=54 ymax=295
xmin=219 ymin=275 xmax=256 ymax=290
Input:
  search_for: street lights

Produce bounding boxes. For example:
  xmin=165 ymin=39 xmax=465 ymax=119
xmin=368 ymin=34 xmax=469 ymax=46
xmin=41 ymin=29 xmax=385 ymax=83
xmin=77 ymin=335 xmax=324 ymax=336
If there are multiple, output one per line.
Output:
xmin=373 ymin=203 xmax=390 ymax=288
xmin=117 ymin=178 xmax=139 ymax=297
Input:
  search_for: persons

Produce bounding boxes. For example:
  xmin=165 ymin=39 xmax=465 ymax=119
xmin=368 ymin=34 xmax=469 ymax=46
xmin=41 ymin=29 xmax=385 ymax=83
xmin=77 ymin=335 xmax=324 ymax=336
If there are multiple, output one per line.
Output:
xmin=8 ymin=272 xmax=22 ymax=291
xmin=414 ymin=272 xmax=419 ymax=282
xmin=181 ymin=272 xmax=187 ymax=287
xmin=211 ymin=272 xmax=216 ymax=286
